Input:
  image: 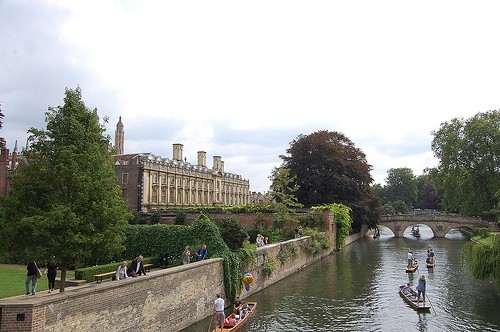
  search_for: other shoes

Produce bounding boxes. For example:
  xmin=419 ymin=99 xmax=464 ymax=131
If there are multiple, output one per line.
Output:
xmin=26 ymin=292 xmax=30 ymax=295
xmin=52 ymin=289 xmax=54 ymax=291
xmin=32 ymin=292 xmax=35 ymax=295
xmin=48 ymin=291 xmax=51 ymax=293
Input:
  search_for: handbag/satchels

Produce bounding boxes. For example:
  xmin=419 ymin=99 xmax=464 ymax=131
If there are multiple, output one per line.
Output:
xmin=128 ymin=272 xmax=137 ymax=277
xmin=112 ymin=275 xmax=116 ymax=280
xmin=36 ymin=269 xmax=42 ymax=278
xmin=44 ymin=269 xmax=48 ymax=277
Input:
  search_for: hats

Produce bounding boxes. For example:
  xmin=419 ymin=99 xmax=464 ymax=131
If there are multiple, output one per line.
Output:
xmin=217 ymin=294 xmax=221 ymax=296
xmin=420 ymin=275 xmax=426 ymax=280
xmin=257 ymin=234 xmax=261 ymax=237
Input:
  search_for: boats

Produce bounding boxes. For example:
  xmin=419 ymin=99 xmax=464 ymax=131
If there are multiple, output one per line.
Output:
xmin=405 ymin=260 xmax=418 ymax=273
xmin=398 ymin=284 xmax=431 ymax=311
xmin=210 ymin=302 xmax=258 ymax=332
xmin=410 ymin=224 xmax=420 ymax=237
xmin=424 ymin=256 xmax=436 ymax=267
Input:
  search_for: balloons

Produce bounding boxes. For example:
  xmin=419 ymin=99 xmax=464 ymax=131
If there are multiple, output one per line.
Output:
xmin=242 ymin=271 xmax=254 ymax=292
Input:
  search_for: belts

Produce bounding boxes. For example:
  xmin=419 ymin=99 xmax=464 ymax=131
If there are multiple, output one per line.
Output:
xmin=216 ymin=311 xmax=224 ymax=312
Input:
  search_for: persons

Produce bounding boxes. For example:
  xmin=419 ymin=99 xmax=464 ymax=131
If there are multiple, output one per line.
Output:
xmin=182 ymin=245 xmax=191 ymax=265
xmin=218 ymin=313 xmax=237 ymax=328
xmin=234 ymin=297 xmax=251 ymax=319
xmin=116 ymin=260 xmax=131 ymax=281
xmin=294 ymin=225 xmax=304 ymax=237
xmin=256 ymin=234 xmax=271 ymax=247
xmin=194 ymin=243 xmax=207 ymax=262
xmin=26 ymin=257 xmax=40 ymax=295
xmin=126 ymin=254 xmax=147 ymax=277
xmin=426 ymin=248 xmax=435 ymax=264
xmin=407 ymin=251 xmax=413 ymax=269
xmin=211 ymin=292 xmax=225 ymax=332
xmin=42 ymin=255 xmax=60 ymax=293
xmin=417 ymin=274 xmax=428 ymax=306
xmin=399 ymin=282 xmax=414 ymax=299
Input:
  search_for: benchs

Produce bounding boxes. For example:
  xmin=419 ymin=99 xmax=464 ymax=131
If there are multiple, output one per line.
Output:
xmin=412 ymin=298 xmax=424 ymax=302
xmin=143 ymin=262 xmax=154 ymax=272
xmin=93 ymin=271 xmax=117 ymax=283
